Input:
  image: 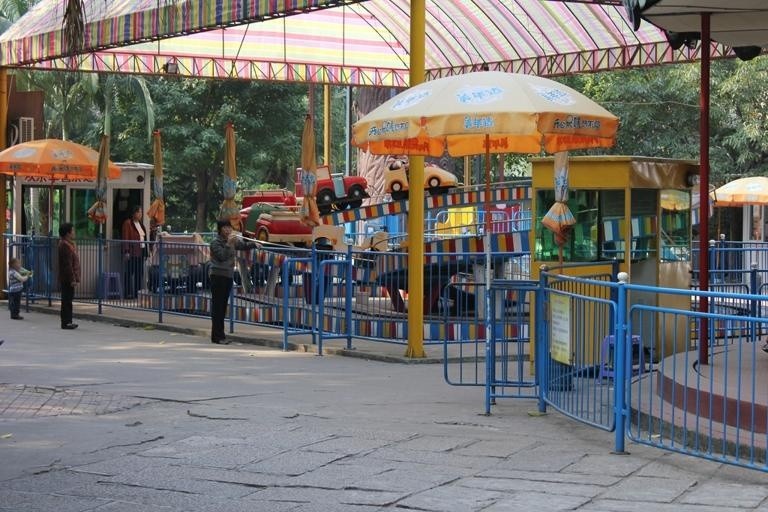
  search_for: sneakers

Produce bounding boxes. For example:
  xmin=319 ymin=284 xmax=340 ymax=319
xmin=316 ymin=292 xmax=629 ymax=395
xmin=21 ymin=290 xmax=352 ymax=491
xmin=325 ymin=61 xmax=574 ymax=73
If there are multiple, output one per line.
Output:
xmin=60 ymin=323 xmax=79 ymax=330
xmin=10 ymin=314 xmax=23 ymax=319
xmin=218 ymin=338 xmax=233 ymax=343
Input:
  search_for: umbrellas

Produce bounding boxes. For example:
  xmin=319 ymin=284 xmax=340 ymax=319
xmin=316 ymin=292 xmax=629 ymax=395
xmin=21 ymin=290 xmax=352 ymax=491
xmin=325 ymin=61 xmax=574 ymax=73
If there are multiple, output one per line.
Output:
xmin=86 ymin=132 xmax=110 ymax=224
xmin=217 ymin=122 xmax=240 ymax=227
xmin=0 ymin=138 xmax=112 ymax=235
xmin=348 ymin=65 xmax=625 ymax=411
xmin=145 ymin=130 xmax=165 ymax=228
xmin=541 ymin=149 xmax=575 ymax=276
xmin=301 ymin=114 xmax=322 ymax=246
xmin=707 ymin=177 xmax=768 ymax=239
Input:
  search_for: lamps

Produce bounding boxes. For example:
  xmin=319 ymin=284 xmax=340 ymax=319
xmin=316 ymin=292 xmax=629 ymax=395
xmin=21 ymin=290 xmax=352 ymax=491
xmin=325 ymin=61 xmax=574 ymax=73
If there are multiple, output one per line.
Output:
xmin=733 ymin=47 xmax=760 ymax=62
xmin=665 ymin=29 xmax=702 ymax=50
xmin=163 ymin=62 xmax=178 ymax=74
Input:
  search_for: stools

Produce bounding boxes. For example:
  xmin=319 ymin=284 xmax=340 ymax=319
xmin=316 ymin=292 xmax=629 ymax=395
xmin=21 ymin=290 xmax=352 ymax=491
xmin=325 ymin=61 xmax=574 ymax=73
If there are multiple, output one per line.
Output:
xmin=596 ymin=336 xmax=645 ymax=384
xmin=97 ymin=272 xmax=124 ymax=300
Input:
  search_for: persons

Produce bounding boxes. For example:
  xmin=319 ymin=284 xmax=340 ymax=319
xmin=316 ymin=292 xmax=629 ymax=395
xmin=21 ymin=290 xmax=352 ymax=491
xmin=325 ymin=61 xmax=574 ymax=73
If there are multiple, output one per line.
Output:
xmin=58 ymin=222 xmax=82 ymax=330
xmin=122 ymin=204 xmax=148 ymax=301
xmin=206 ymin=217 xmax=265 ymax=344
xmin=8 ymin=257 xmax=32 ymax=320
